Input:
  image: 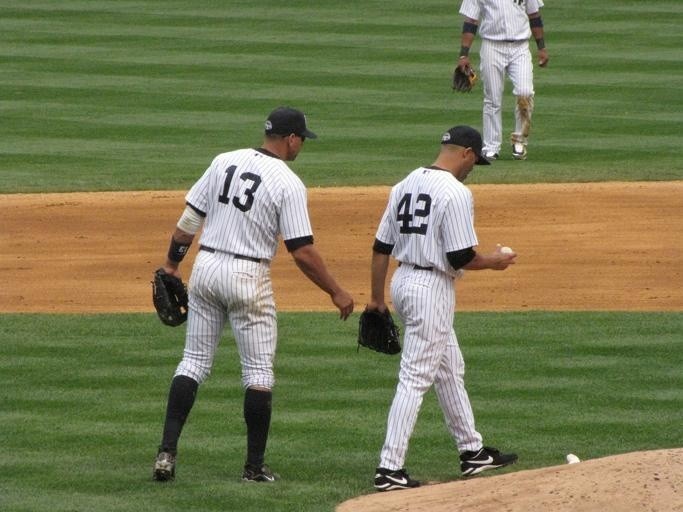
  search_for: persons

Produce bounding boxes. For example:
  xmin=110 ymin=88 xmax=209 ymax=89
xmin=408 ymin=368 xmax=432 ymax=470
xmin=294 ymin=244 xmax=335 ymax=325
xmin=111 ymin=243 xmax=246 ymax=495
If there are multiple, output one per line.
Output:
xmin=451 ymin=0 xmax=549 ymax=160
xmin=358 ymin=122 xmax=518 ymax=493
xmin=143 ymin=103 xmax=356 ymax=486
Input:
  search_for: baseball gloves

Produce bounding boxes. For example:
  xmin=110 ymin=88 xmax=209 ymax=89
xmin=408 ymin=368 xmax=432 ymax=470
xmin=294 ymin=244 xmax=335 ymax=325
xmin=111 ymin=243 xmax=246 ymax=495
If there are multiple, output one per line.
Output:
xmin=358 ymin=304 xmax=401 ymax=355
xmin=150 ymin=268 xmax=188 ymax=326
xmin=452 ymin=63 xmax=477 ymax=93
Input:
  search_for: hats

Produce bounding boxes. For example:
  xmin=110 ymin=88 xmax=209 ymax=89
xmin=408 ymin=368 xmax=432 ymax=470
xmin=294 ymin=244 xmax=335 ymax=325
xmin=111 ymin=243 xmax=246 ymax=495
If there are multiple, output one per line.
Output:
xmin=441 ymin=126 xmax=491 ymax=165
xmin=265 ymin=106 xmax=318 ymax=139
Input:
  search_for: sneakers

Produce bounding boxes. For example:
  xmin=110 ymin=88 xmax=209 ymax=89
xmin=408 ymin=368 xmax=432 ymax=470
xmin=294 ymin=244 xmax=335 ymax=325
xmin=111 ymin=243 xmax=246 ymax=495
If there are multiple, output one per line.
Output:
xmin=511 ymin=141 xmax=528 ymax=159
xmin=373 ymin=468 xmax=420 ymax=492
xmin=459 ymin=447 xmax=518 ymax=477
xmin=242 ymin=462 xmax=281 ymax=483
xmin=482 ymin=150 xmax=499 ymax=162
xmin=152 ymin=451 xmax=176 ymax=480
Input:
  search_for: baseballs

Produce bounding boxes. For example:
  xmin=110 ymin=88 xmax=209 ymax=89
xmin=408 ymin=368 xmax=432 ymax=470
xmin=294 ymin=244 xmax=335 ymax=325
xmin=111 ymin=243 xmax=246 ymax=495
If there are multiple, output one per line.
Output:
xmin=566 ymin=454 xmax=579 ymax=466
xmin=500 ymin=247 xmax=511 ymax=254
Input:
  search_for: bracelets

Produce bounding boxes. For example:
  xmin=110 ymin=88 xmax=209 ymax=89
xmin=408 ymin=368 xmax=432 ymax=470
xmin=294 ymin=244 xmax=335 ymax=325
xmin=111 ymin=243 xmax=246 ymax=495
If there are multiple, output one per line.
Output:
xmin=535 ymin=37 xmax=544 ymax=50
xmin=459 ymin=45 xmax=469 ymax=57
xmin=165 ymin=234 xmax=192 ymax=264
